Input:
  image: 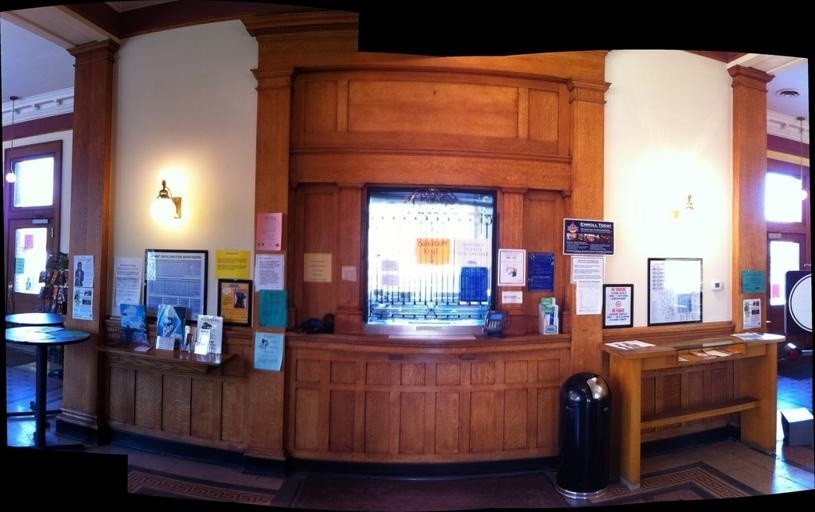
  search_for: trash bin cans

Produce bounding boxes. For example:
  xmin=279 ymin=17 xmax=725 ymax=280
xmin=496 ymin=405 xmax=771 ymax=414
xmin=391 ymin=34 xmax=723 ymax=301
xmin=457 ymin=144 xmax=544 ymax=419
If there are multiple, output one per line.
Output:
xmin=556 ymin=372 xmax=612 ymax=500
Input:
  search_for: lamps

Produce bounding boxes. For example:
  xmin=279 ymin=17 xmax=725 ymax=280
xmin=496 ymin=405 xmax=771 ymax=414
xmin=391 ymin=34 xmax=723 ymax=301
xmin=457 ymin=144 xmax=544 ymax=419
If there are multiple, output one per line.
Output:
xmin=793 ymin=114 xmax=809 ymax=204
xmin=153 ymin=166 xmax=190 ymax=225
xmin=5 ymin=94 xmax=23 ymax=183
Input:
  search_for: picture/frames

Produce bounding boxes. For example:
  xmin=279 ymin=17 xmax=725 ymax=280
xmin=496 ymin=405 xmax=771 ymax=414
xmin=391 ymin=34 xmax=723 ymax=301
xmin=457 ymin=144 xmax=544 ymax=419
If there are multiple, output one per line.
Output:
xmin=141 ymin=249 xmax=208 ymax=324
xmin=602 ymin=284 xmax=634 ymax=328
xmin=646 ymin=257 xmax=705 ymax=327
xmin=216 ymin=277 xmax=253 ymax=328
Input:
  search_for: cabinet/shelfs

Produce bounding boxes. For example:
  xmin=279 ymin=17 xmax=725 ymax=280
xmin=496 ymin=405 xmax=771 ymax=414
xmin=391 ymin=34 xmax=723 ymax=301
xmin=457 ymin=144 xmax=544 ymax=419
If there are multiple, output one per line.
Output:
xmin=602 ymin=333 xmax=787 ymax=491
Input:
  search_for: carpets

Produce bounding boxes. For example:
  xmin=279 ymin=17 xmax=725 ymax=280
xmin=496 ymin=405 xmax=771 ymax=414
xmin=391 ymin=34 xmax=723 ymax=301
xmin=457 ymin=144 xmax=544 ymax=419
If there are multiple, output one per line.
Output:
xmin=282 ymin=470 xmax=574 ymax=507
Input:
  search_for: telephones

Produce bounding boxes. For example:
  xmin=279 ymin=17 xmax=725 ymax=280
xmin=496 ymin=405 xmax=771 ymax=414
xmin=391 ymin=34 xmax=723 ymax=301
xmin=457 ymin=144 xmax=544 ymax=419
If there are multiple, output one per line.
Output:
xmin=484 ymin=311 xmax=507 ymax=333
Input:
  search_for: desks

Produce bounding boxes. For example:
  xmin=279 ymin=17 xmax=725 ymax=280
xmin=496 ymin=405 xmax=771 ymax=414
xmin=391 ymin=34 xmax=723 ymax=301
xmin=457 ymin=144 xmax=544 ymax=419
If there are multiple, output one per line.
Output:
xmin=6 ymin=326 xmax=90 ymax=446
xmin=6 ymin=312 xmax=64 ymax=427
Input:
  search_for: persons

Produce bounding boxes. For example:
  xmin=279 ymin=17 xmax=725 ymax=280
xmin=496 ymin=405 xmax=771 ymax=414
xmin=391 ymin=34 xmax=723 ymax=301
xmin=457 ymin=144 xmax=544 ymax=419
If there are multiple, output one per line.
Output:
xmin=163 ymin=318 xmax=175 ymax=336
xmin=75 ymin=261 xmax=86 ymax=288
